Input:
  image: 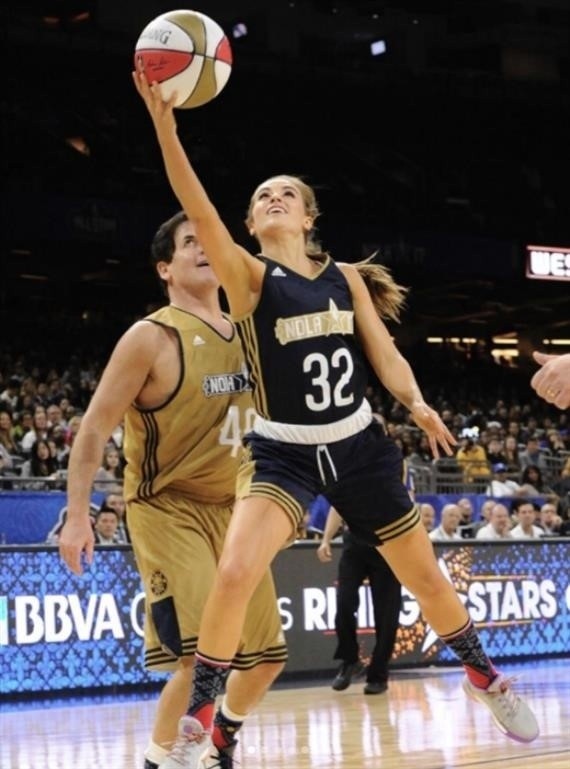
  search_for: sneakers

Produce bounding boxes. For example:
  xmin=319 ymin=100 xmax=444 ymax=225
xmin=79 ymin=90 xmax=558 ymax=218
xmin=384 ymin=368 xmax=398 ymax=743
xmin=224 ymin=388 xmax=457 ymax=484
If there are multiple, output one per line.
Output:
xmin=158 ymin=715 xmax=211 ymax=769
xmin=197 ymin=739 xmax=239 ymax=769
xmin=462 ymin=672 xmax=538 ymax=742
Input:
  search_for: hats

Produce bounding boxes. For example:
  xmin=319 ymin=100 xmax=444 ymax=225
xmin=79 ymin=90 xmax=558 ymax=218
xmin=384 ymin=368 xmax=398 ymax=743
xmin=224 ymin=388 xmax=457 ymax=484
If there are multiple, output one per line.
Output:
xmin=494 ymin=463 xmax=505 ymax=472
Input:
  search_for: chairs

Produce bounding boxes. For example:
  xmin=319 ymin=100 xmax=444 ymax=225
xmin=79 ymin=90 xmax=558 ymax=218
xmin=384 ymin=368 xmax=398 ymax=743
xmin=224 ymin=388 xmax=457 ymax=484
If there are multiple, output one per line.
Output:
xmin=408 ymin=445 xmax=570 ymax=494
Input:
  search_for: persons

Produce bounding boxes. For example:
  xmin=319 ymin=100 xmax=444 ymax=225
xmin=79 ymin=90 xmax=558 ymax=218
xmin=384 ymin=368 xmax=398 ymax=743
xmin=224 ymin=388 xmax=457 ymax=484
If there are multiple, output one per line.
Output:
xmin=130 ymin=70 xmax=539 ymax=769
xmin=59 ymin=212 xmax=288 ymax=769
xmin=297 ymin=386 xmax=570 ymax=539
xmin=0 ymin=353 xmax=130 ymax=545
xmin=317 ymin=413 xmax=402 ymax=695
xmin=530 ymin=351 xmax=570 ymax=410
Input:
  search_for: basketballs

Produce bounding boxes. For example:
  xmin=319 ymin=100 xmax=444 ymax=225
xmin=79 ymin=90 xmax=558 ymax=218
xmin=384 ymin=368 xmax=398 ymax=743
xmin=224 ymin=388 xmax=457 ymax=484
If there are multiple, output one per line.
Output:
xmin=132 ymin=10 xmax=233 ymax=110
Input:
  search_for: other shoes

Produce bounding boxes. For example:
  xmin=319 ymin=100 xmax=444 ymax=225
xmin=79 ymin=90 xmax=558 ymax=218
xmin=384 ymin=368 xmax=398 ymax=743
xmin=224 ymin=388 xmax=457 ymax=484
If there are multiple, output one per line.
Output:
xmin=364 ymin=679 xmax=389 ymax=694
xmin=332 ymin=660 xmax=363 ymax=690
